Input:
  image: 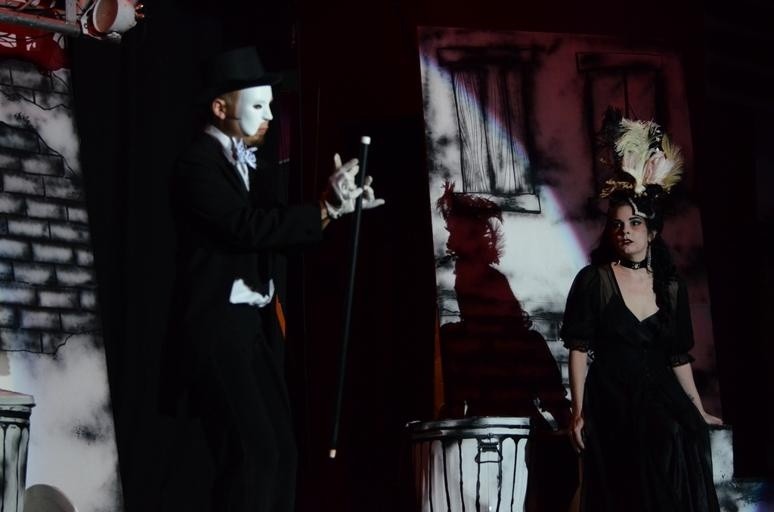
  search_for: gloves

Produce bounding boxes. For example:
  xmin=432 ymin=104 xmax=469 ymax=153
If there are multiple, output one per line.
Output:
xmin=322 ymin=153 xmax=385 ymax=220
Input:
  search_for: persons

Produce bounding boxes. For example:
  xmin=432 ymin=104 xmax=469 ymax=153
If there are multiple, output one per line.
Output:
xmin=157 ymin=83 xmax=385 ymax=512
xmin=559 ymin=190 xmax=727 ymax=512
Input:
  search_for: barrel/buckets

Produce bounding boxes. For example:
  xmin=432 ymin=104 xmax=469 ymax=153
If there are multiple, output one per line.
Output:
xmin=0 ymin=390 xmax=33 ymax=511
xmin=405 ymin=416 xmax=532 ymax=510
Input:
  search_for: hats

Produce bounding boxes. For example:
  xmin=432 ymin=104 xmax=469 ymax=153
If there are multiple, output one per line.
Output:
xmin=197 ymin=47 xmax=284 ymax=99
